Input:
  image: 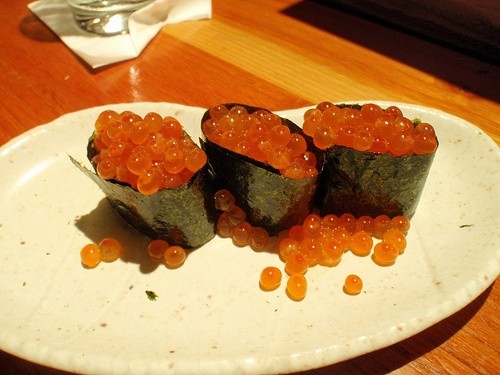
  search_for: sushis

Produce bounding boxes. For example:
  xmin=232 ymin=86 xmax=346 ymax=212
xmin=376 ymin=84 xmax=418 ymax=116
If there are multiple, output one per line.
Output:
xmin=67 ymin=105 xmax=220 ymax=249
xmin=197 ymin=99 xmax=322 ymax=237
xmin=300 ymin=99 xmax=439 ymax=226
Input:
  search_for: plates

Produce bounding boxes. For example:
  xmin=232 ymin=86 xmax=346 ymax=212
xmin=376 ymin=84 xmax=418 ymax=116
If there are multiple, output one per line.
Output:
xmin=1 ymin=100 xmax=500 ymax=374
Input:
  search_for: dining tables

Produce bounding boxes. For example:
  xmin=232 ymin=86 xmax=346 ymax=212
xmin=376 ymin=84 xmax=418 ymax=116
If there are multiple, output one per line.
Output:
xmin=0 ymin=0 xmax=500 ymax=375
